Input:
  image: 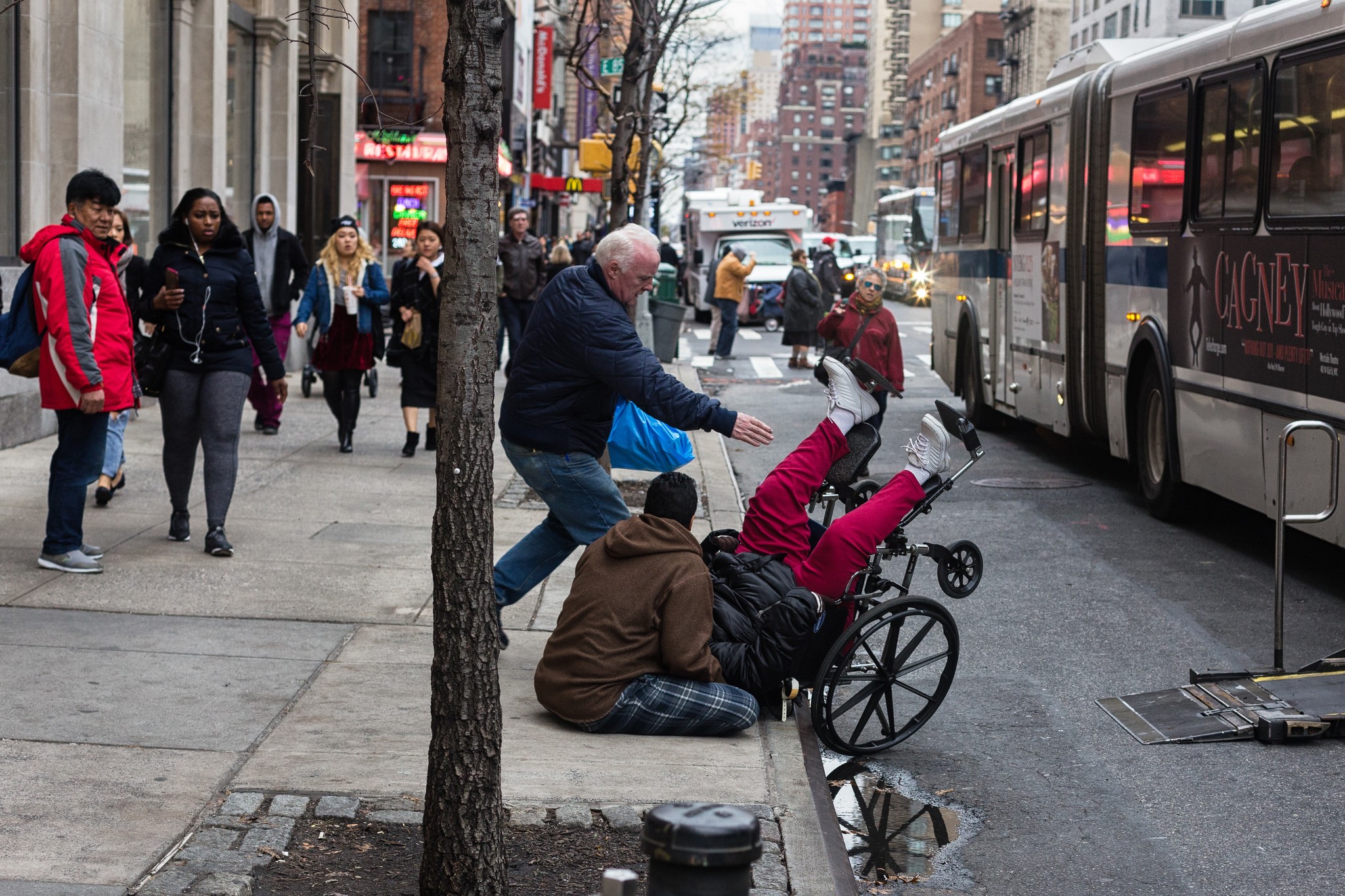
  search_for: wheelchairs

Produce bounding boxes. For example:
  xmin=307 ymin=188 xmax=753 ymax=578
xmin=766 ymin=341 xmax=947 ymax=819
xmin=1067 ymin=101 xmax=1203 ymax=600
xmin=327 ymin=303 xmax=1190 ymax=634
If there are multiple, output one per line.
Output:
xmin=740 ymin=354 xmax=988 ymax=757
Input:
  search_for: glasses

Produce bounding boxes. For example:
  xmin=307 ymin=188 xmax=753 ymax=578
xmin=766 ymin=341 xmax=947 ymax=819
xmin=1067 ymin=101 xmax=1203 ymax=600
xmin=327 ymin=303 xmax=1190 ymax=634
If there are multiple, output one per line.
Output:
xmin=864 ymin=280 xmax=882 ymax=291
xmin=801 ymin=255 xmax=806 ymax=259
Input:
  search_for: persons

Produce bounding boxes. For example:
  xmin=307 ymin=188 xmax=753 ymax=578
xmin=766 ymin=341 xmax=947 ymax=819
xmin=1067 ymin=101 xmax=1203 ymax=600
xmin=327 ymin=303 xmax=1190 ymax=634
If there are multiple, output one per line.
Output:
xmin=660 ymin=235 xmax=679 ymax=266
xmin=817 ymin=267 xmax=904 ymax=476
xmin=496 ymin=207 xmax=598 ymax=369
xmin=715 ymin=247 xmax=758 ymax=360
xmin=703 ymin=245 xmax=732 ymax=354
xmin=533 ymin=472 xmax=758 ymax=737
xmin=136 ymin=188 xmax=288 ymax=556
xmin=390 ymin=221 xmax=446 ymax=457
xmin=240 ymin=193 xmax=308 ymax=434
xmin=781 ymin=249 xmax=821 ymax=369
xmin=96 ymin=208 xmax=148 ymax=507
xmin=710 ymin=355 xmax=951 ymax=699
xmin=492 ymin=223 xmax=773 ymax=650
xmin=19 ymin=169 xmax=144 ymax=572
xmin=813 ymin=237 xmax=844 ymax=317
xmin=295 ymin=216 xmax=392 ymax=454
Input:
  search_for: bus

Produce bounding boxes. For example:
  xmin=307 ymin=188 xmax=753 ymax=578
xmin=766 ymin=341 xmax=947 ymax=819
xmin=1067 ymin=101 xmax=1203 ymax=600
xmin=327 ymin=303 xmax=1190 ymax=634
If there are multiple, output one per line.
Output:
xmin=901 ymin=0 xmax=1345 ymax=555
xmin=868 ymin=185 xmax=935 ymax=305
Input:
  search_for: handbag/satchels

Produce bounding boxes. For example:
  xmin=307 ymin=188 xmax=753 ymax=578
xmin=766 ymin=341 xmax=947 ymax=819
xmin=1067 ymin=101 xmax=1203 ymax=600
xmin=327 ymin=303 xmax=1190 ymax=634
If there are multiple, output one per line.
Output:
xmin=371 ymin=305 xmax=385 ymax=361
xmin=401 ymin=306 xmax=422 ymax=349
xmin=606 ymin=397 xmax=695 ymax=474
xmin=813 ymin=347 xmax=851 ymax=387
xmin=131 ymin=335 xmax=160 ymax=399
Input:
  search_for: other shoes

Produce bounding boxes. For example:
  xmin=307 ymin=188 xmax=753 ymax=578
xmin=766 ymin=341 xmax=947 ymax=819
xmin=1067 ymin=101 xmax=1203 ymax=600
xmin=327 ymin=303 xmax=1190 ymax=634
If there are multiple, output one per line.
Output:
xmin=497 ymin=618 xmax=510 ymax=653
xmin=861 ymin=466 xmax=870 ymax=476
xmin=262 ymin=423 xmax=277 ymax=433
xmin=797 ymin=357 xmax=815 ymax=369
xmin=111 ymin=472 xmax=126 ymax=499
xmin=94 ymin=485 xmax=110 ymax=508
xmin=254 ymin=411 xmax=264 ymax=430
xmin=788 ymin=357 xmax=799 ymax=368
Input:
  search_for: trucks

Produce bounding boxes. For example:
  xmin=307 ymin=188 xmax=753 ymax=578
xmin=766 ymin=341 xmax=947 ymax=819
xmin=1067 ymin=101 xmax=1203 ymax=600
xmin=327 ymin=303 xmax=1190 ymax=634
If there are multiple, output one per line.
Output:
xmin=687 ymin=195 xmax=813 ymax=321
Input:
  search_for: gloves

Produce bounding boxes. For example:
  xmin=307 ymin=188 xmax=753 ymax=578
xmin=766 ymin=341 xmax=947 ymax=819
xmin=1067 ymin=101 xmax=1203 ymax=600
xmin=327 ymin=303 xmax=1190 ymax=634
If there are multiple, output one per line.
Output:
xmin=712 ymin=534 xmax=740 ymax=551
xmin=811 ymin=591 xmax=837 ymax=618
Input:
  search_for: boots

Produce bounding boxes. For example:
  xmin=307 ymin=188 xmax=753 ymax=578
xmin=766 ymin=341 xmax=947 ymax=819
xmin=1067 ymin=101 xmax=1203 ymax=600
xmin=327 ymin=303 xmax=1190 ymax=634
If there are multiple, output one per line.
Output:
xmin=425 ymin=423 xmax=436 ymax=451
xmin=401 ymin=430 xmax=421 ymax=457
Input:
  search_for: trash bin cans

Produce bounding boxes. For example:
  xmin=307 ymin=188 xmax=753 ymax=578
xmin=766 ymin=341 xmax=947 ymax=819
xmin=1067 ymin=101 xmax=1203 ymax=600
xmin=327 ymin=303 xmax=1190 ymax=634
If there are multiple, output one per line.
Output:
xmin=653 ymin=300 xmax=687 ymax=364
xmin=651 ymin=262 xmax=677 ymax=306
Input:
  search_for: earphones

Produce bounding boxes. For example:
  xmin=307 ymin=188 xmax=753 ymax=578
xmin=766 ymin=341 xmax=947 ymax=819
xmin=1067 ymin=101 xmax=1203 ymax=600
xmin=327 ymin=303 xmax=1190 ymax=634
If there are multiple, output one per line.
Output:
xmin=185 ymin=218 xmax=189 ymax=225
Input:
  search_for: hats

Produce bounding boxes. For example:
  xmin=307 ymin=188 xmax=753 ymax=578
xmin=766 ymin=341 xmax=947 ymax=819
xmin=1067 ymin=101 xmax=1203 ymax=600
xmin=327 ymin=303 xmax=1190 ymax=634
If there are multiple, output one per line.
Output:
xmin=331 ymin=215 xmax=359 ymax=235
xmin=822 ymin=237 xmax=838 ymax=245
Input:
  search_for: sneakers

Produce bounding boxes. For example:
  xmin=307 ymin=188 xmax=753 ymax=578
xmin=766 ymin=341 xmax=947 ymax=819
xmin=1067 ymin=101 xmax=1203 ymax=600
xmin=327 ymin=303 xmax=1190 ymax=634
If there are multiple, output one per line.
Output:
xmin=36 ymin=549 xmax=103 ymax=574
xmin=900 ymin=413 xmax=951 ymax=477
xmin=203 ymin=525 xmax=235 ymax=556
xmin=167 ymin=509 xmax=191 ymax=541
xmin=81 ymin=542 xmax=103 ymax=560
xmin=821 ymin=355 xmax=879 ymax=424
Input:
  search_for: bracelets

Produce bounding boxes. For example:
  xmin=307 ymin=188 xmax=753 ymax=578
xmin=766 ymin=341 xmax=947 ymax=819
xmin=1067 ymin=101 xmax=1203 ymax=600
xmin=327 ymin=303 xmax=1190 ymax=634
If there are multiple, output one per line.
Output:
xmin=429 ymin=272 xmax=439 ymax=279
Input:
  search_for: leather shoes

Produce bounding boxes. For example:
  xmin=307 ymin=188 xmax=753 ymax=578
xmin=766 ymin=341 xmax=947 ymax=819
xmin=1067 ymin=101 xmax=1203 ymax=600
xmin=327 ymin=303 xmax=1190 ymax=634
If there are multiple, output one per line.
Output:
xmin=338 ymin=426 xmax=353 ymax=453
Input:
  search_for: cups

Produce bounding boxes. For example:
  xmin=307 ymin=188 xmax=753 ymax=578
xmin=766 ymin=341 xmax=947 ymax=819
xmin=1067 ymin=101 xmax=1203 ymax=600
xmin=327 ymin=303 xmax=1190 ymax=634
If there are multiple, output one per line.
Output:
xmin=342 ymin=286 xmax=359 ymax=315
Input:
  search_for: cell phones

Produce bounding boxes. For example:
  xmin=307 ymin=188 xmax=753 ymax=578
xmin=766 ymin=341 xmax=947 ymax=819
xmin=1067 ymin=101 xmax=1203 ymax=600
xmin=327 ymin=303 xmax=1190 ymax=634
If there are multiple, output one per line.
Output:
xmin=165 ymin=268 xmax=180 ymax=290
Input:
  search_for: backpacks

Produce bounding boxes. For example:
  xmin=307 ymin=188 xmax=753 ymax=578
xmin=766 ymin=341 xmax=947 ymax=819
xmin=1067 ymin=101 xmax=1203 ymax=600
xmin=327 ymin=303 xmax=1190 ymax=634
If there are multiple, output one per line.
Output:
xmin=0 ymin=232 xmax=92 ymax=380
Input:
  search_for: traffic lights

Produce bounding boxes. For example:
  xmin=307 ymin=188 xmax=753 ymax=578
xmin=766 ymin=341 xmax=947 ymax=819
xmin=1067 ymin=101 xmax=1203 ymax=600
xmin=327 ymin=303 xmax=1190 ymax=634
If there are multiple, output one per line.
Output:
xmin=603 ymin=176 xmax=614 ymax=201
xmin=749 ymin=160 xmax=763 ymax=181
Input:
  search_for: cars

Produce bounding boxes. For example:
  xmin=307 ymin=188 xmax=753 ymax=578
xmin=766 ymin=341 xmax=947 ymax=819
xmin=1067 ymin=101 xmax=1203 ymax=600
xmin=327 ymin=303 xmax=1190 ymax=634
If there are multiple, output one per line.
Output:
xmin=805 ymin=232 xmax=876 ymax=296
